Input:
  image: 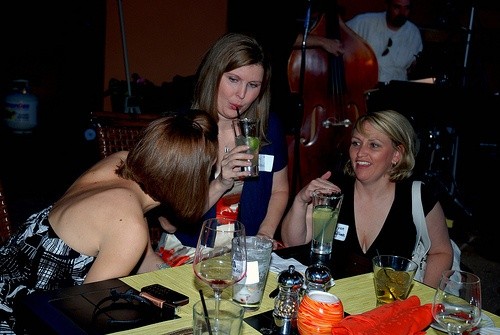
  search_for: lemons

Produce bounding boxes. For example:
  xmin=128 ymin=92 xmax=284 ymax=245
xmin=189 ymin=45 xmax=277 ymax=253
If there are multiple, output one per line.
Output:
xmin=248 ymin=136 xmax=259 ymax=150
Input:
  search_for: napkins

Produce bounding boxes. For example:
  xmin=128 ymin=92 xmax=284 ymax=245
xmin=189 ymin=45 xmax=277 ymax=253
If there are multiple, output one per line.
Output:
xmin=269 ymin=251 xmax=309 ymax=275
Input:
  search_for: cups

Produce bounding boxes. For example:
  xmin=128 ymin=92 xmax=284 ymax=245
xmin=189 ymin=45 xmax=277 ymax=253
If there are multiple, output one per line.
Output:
xmin=371 ymin=254 xmax=419 ymax=309
xmin=230 ymin=236 xmax=273 ymax=312
xmin=232 ymin=118 xmax=261 ymax=177
xmin=311 ymin=187 xmax=345 ymax=255
xmin=193 ymin=298 xmax=246 ymax=335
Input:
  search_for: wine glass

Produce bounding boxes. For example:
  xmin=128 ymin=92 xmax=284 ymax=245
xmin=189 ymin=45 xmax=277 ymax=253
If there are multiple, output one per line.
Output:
xmin=193 ymin=218 xmax=247 ymax=335
xmin=431 ymin=269 xmax=482 ymax=335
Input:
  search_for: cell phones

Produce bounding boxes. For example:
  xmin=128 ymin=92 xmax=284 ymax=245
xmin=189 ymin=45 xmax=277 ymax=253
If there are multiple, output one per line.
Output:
xmin=142 ymin=283 xmax=189 ymax=306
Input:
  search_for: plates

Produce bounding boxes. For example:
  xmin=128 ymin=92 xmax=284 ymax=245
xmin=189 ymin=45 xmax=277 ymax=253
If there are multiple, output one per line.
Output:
xmin=429 ymin=306 xmax=494 ymax=333
xmin=471 ymin=327 xmax=500 ymax=335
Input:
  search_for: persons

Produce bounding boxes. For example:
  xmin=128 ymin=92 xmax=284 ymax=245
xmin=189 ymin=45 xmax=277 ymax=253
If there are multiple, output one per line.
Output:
xmin=0 ymin=113 xmax=218 ymax=335
xmin=280 ymin=110 xmax=453 ymax=290
xmin=344 ymin=0 xmax=424 ymax=83
xmin=253 ymin=0 xmax=345 ymax=140
xmin=154 ymin=32 xmax=290 ymax=259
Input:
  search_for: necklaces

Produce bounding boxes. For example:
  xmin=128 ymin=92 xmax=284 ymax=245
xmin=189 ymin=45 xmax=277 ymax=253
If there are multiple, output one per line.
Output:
xmin=224 ymin=147 xmax=231 ymax=153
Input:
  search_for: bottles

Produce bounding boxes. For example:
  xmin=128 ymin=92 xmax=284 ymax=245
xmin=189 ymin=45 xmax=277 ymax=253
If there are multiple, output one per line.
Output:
xmin=273 ymin=264 xmax=303 ymax=329
xmin=304 ymin=261 xmax=332 ymax=294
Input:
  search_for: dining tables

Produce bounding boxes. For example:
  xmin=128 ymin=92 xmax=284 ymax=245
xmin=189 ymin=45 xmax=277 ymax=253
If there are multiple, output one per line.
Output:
xmin=10 ymin=242 xmax=500 ymax=335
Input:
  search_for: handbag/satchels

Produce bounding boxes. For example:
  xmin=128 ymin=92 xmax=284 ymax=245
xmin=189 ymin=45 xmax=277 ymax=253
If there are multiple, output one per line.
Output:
xmin=405 ymin=180 xmax=462 ymax=296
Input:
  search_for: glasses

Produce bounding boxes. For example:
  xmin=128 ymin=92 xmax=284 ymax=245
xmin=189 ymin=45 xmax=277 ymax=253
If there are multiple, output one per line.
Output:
xmin=382 ymin=38 xmax=393 ymax=56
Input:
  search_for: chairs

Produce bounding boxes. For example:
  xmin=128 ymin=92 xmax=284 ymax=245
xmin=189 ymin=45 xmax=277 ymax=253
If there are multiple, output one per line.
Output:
xmin=90 ymin=110 xmax=161 ymax=156
